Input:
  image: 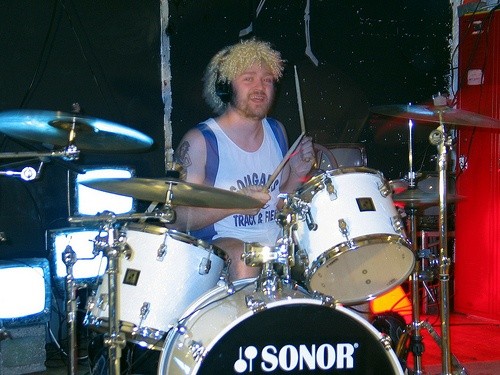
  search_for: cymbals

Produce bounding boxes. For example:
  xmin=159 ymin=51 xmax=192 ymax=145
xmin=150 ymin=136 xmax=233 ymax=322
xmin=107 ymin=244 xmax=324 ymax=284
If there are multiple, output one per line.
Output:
xmin=390 ymin=188 xmax=469 ymax=201
xmin=369 ymin=104 xmax=500 ymax=129
xmin=79 ymin=179 xmax=262 ymax=211
xmin=7 ymin=135 xmax=87 ymax=175
xmin=0 ymin=111 xmax=154 ymax=153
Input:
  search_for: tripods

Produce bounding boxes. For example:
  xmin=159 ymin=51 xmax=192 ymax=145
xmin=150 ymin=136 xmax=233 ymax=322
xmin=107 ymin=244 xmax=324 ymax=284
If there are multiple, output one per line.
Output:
xmin=394 ymin=119 xmax=466 ymax=375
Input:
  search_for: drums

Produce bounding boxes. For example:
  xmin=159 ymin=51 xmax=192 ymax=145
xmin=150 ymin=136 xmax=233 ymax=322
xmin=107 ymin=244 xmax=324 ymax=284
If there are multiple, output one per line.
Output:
xmin=82 ymin=219 xmax=229 ymax=353
xmin=158 ymin=275 xmax=405 ymax=375
xmin=288 ymin=165 xmax=416 ymax=307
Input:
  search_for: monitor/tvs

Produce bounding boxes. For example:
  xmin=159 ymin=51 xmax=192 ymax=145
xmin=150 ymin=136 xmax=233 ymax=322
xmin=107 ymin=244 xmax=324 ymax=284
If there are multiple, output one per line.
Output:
xmin=0 ymin=167 xmax=139 ymax=327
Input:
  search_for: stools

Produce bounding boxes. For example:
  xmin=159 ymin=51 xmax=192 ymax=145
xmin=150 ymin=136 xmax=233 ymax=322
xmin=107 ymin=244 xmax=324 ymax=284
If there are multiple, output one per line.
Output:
xmin=416 ymin=230 xmax=454 ymax=314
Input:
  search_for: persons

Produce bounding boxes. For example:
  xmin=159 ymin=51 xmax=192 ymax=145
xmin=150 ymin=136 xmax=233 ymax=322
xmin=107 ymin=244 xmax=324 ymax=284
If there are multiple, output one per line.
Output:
xmin=166 ymin=38 xmax=318 ymax=284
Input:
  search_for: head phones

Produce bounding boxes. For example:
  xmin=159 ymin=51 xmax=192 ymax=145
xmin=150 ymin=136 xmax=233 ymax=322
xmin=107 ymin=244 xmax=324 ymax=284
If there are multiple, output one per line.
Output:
xmin=215 ymin=50 xmax=233 ymax=104
